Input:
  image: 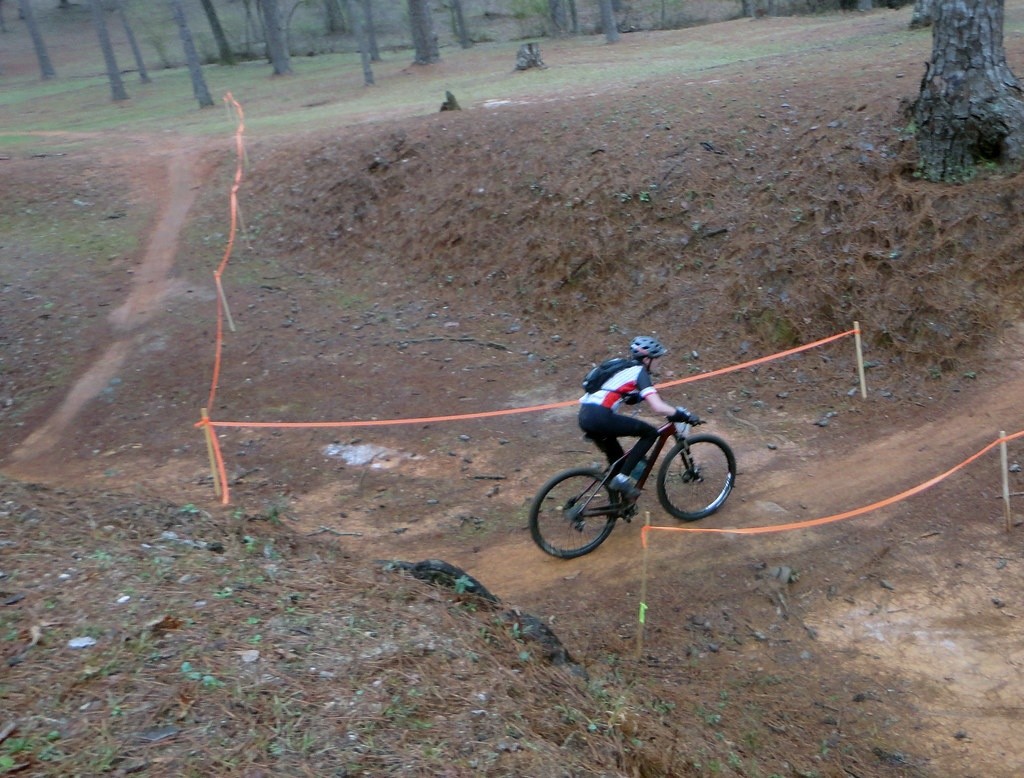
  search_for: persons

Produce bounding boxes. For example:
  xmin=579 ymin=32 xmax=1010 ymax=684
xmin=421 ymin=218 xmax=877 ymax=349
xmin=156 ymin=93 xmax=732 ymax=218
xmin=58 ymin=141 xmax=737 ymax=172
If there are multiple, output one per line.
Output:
xmin=578 ymin=335 xmax=692 ymax=497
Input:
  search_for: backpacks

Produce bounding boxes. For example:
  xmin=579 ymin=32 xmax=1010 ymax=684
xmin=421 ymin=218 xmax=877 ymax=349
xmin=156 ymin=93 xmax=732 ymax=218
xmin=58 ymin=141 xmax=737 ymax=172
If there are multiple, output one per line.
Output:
xmin=583 ymin=357 xmax=641 ymax=396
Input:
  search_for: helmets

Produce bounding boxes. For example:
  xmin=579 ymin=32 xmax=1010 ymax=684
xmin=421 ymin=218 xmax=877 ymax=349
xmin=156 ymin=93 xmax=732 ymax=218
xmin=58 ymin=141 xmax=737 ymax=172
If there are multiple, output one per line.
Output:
xmin=629 ymin=335 xmax=668 ymax=359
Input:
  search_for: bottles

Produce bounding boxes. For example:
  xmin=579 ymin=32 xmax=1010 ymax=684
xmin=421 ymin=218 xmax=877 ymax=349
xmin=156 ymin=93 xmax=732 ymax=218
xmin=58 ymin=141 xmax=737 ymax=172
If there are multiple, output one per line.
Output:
xmin=628 ymin=456 xmax=648 ymax=486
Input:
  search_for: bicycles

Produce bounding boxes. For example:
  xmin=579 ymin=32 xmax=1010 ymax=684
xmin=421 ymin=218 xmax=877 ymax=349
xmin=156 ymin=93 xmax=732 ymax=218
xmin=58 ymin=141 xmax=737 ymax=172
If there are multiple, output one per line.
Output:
xmin=529 ymin=406 xmax=737 ymax=558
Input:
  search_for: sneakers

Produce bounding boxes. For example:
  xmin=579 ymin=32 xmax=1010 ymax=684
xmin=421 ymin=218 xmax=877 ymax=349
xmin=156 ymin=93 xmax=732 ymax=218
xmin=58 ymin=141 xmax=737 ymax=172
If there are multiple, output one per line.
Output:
xmin=609 ymin=476 xmax=641 ymax=497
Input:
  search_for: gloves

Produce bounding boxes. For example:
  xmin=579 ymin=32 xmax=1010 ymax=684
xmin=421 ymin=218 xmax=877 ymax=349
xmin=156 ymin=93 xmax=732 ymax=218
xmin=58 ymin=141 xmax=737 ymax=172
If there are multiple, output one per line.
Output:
xmin=688 ymin=413 xmax=702 ymax=427
